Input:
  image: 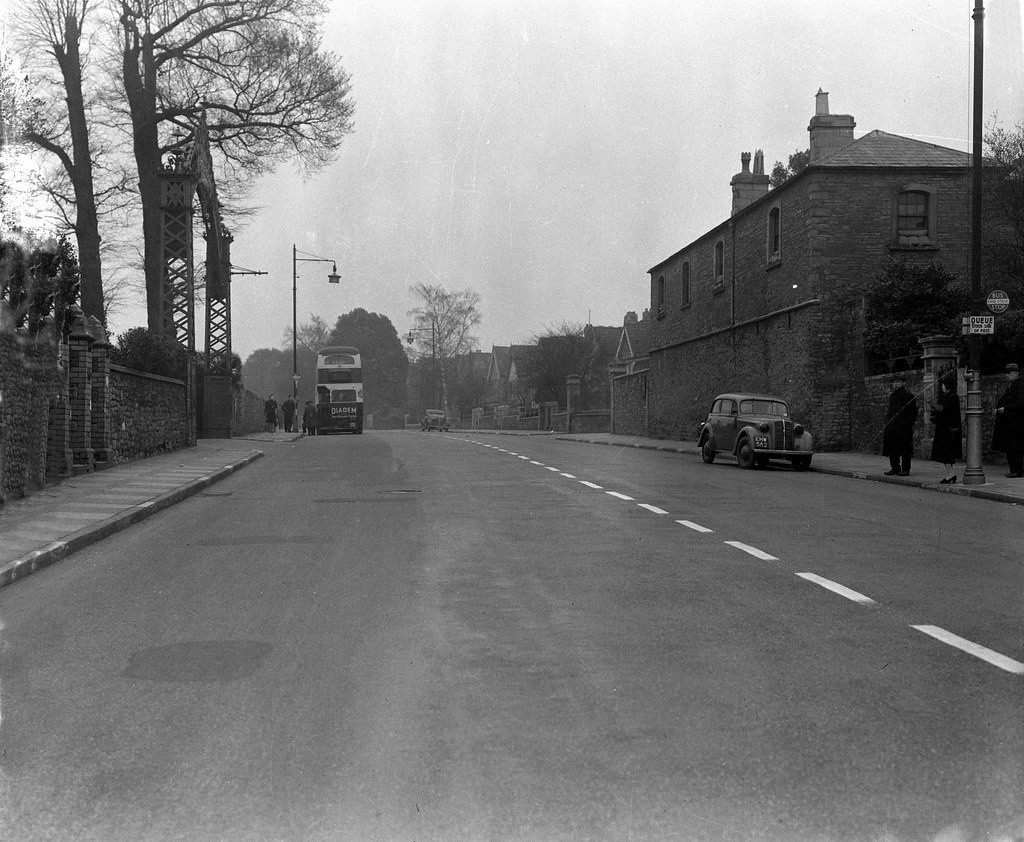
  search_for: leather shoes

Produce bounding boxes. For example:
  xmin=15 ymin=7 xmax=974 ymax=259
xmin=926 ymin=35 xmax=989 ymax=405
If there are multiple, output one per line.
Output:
xmin=884 ymin=470 xmax=901 ymax=475
xmin=899 ymin=471 xmax=909 ymax=476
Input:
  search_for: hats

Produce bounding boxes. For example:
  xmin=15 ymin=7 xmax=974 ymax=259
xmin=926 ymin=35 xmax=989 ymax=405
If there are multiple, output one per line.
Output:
xmin=891 ymin=372 xmax=906 ymax=381
xmin=1005 ymin=363 xmax=1019 ymax=371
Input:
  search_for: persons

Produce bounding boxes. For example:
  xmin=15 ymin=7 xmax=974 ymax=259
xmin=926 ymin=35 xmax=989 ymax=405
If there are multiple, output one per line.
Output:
xmin=264 ymin=394 xmax=278 ymax=435
xmin=930 ymin=378 xmax=961 ymax=484
xmin=277 ymin=392 xmax=331 ymax=437
xmin=994 ymin=364 xmax=1024 ymax=477
xmin=882 ymin=375 xmax=917 ymax=477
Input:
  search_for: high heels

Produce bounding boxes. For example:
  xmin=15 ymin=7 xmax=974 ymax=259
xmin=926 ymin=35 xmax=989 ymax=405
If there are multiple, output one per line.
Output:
xmin=940 ymin=475 xmax=957 ymax=484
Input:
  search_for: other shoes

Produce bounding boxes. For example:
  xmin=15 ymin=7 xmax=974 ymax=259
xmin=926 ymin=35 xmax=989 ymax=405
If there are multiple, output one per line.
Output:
xmin=1005 ymin=473 xmax=1023 ymax=477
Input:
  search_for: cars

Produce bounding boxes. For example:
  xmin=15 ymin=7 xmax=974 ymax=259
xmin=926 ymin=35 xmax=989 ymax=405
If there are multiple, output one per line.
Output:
xmin=420 ymin=408 xmax=449 ymax=432
xmin=699 ymin=391 xmax=814 ymax=472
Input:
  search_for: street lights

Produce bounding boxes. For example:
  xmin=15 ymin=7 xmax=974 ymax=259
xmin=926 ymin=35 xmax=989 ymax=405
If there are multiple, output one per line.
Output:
xmin=407 ymin=320 xmax=436 ymax=408
xmin=291 ymin=244 xmax=342 ymax=433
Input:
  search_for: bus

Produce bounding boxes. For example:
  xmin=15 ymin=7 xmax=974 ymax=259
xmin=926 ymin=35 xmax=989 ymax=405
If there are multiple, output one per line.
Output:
xmin=312 ymin=345 xmax=364 ymax=434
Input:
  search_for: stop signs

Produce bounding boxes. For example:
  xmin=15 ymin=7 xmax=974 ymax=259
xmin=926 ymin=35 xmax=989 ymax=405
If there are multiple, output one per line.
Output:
xmin=986 ymin=291 xmax=1011 ymax=315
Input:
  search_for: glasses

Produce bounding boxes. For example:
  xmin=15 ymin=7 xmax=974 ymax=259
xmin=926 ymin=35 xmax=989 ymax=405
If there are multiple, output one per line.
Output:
xmin=1005 ymin=372 xmax=1011 ymax=374
